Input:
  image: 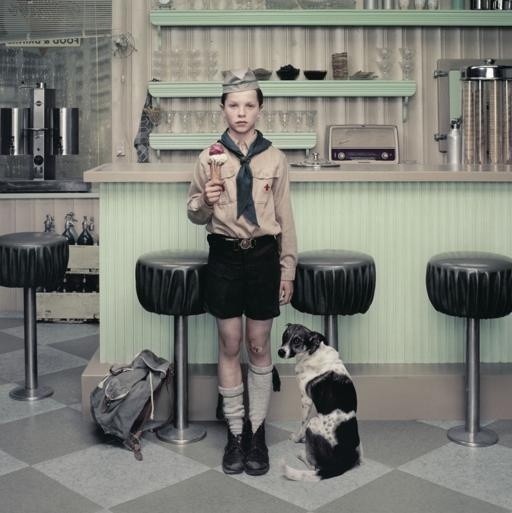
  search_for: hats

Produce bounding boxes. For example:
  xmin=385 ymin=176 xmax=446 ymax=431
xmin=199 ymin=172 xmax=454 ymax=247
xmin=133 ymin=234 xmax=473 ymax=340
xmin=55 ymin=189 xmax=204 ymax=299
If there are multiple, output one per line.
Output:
xmin=221 ymin=67 xmax=260 ymax=94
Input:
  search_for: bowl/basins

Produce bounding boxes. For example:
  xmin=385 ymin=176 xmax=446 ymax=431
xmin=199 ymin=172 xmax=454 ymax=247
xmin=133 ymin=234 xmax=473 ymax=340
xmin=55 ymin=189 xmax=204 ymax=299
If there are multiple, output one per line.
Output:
xmin=252 ymin=69 xmax=273 ymax=81
xmin=276 ymin=69 xmax=300 ymax=80
xmin=303 ymin=70 xmax=327 ymax=80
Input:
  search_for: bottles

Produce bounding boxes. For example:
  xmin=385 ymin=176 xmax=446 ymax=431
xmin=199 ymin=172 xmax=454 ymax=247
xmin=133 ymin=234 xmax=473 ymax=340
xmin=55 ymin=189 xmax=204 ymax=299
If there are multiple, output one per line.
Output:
xmin=44 ymin=211 xmax=97 ymax=246
xmin=446 ymin=118 xmax=463 ymax=166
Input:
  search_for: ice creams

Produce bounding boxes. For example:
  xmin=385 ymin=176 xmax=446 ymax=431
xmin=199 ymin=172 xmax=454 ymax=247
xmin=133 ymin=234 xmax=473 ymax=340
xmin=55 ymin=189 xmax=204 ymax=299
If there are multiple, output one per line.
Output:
xmin=208 ymin=143 xmax=227 ymax=186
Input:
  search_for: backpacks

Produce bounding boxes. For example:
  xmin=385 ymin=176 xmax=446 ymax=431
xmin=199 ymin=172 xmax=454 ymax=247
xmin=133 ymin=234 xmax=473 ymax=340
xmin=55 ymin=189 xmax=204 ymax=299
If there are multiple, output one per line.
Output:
xmin=89 ymin=350 xmax=175 ymax=461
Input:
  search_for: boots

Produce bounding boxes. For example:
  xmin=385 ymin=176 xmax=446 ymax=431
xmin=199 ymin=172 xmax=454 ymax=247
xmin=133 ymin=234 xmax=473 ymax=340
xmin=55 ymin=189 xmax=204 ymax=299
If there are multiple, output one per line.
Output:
xmin=222 ymin=419 xmax=270 ymax=476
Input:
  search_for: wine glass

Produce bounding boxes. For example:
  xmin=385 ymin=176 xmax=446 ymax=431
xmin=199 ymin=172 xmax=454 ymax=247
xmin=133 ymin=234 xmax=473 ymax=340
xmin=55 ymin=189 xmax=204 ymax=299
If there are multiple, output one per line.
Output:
xmin=151 ymin=48 xmax=219 ymax=83
xmin=162 ymin=110 xmax=318 ymax=133
xmin=376 ymin=47 xmax=416 ymax=81
xmin=180 ymin=0 xmax=265 ymax=10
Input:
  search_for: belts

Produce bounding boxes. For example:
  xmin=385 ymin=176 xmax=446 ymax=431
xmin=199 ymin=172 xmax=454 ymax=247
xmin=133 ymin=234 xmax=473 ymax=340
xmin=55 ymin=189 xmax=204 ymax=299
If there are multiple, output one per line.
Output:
xmin=230 ymin=239 xmax=257 ymax=252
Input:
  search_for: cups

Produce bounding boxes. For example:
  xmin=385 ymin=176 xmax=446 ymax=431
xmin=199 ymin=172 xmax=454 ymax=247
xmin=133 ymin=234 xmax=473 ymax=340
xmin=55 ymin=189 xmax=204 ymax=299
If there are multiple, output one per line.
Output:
xmin=451 ymin=1 xmax=466 ymax=10
xmin=363 ymin=0 xmax=440 ymax=9
xmin=470 ymin=0 xmax=512 ymax=10
xmin=332 ymin=50 xmax=349 ymax=80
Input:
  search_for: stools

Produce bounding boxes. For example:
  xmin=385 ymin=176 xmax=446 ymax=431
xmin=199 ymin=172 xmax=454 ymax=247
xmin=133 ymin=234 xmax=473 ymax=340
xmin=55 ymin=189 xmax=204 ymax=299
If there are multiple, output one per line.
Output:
xmin=426 ymin=250 xmax=510 ymax=447
xmin=134 ymin=248 xmax=210 ymax=444
xmin=292 ymin=249 xmax=376 ymax=350
xmin=0 ymin=231 xmax=68 ymax=404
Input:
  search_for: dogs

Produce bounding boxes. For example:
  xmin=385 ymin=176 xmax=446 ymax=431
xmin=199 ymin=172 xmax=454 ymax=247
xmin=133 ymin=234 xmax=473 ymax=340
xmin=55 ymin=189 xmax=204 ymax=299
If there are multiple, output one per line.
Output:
xmin=277 ymin=321 xmax=363 ymax=484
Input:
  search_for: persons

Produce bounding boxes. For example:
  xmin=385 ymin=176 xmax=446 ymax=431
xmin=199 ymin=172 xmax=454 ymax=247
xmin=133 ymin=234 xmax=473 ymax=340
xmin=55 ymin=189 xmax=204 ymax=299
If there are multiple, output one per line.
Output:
xmin=187 ymin=68 xmax=298 ymax=475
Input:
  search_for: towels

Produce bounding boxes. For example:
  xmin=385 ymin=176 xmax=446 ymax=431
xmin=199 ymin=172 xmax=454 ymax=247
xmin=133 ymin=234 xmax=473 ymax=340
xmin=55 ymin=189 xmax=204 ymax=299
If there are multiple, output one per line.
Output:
xmin=133 ymin=78 xmax=160 ymax=164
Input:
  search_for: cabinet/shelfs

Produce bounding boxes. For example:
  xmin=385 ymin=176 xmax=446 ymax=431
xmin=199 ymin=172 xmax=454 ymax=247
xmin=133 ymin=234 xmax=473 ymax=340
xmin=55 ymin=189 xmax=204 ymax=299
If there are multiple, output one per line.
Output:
xmin=35 ymin=241 xmax=99 ymax=323
xmin=145 ymin=8 xmax=512 ymax=158
